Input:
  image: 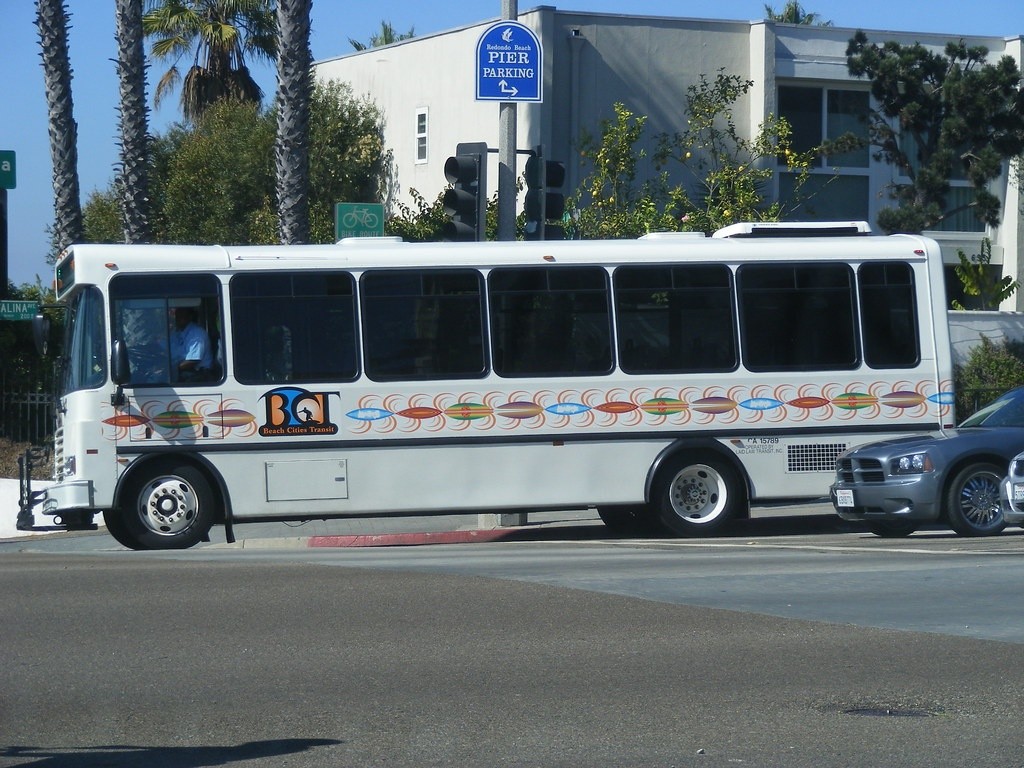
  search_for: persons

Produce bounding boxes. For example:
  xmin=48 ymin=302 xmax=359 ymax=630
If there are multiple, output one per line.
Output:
xmin=156 ymin=309 xmax=212 ymax=382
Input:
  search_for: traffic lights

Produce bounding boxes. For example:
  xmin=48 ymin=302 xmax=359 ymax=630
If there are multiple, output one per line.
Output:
xmin=442 ymin=142 xmax=489 ymax=243
xmin=523 ymin=155 xmax=568 ymax=242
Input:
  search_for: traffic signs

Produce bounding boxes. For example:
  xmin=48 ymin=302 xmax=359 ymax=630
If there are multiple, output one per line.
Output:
xmin=476 ymin=19 xmax=544 ymax=104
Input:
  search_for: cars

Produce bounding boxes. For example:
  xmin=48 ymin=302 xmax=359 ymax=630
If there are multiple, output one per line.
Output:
xmin=1000 ymin=449 xmax=1024 ymax=531
xmin=828 ymin=385 xmax=1024 ymax=539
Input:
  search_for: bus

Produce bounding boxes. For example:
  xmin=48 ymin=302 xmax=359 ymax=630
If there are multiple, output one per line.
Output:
xmin=14 ymin=219 xmax=957 ymax=550
